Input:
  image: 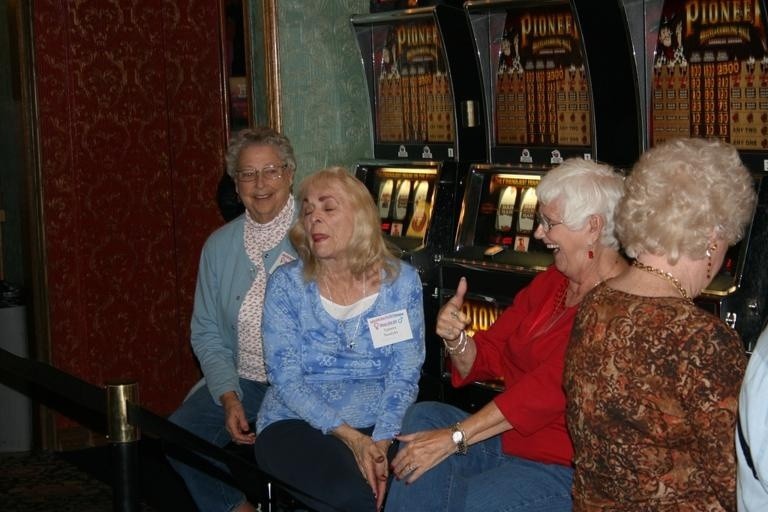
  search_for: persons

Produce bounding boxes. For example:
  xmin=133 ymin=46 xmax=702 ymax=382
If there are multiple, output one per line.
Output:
xmin=734 ymin=324 xmax=768 ymax=512
xmin=382 ymin=157 xmax=626 ymax=512
xmin=162 ymin=127 xmax=303 ymax=512
xmin=254 ymin=166 xmax=426 ymax=512
xmin=561 ymin=137 xmax=758 ymax=512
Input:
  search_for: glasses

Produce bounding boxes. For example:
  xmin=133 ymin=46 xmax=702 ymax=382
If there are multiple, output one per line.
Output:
xmin=536 ymin=213 xmax=563 ymax=232
xmin=233 ymin=164 xmax=288 ymax=184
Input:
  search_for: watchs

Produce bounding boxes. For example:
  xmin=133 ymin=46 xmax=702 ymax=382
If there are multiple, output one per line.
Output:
xmin=451 ymin=422 xmax=468 ymax=454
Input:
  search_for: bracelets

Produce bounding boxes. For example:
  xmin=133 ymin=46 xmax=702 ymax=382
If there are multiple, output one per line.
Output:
xmin=442 ymin=330 xmax=468 ymax=355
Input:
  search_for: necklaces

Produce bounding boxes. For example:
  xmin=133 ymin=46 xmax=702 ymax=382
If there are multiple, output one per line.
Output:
xmin=632 ymin=261 xmax=692 ymax=301
xmin=541 ymin=256 xmax=621 ymax=334
xmin=321 ymin=271 xmax=366 ymax=348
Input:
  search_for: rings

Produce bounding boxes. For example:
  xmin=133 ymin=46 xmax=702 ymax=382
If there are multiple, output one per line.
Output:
xmin=409 ymin=464 xmax=416 ymax=470
